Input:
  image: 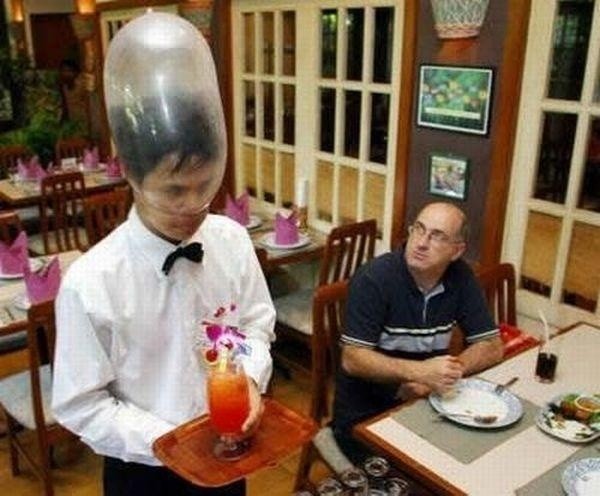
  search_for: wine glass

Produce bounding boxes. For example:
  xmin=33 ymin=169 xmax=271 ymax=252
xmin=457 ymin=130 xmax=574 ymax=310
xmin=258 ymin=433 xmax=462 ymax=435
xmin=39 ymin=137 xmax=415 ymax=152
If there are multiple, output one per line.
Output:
xmin=199 ymin=353 xmax=252 ymax=462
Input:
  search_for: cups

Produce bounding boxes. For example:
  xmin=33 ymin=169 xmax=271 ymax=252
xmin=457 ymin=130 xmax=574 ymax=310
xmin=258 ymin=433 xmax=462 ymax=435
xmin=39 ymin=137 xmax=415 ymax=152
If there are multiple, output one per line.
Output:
xmin=61 ymin=156 xmax=78 ymax=187
xmin=290 ymin=454 xmax=414 ymax=496
xmin=535 ymin=332 xmax=567 ymax=385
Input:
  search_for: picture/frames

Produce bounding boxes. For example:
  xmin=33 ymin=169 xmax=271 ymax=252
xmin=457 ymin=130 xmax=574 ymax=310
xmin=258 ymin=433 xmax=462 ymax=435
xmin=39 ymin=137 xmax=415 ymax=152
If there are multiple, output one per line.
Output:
xmin=414 ymin=60 xmax=496 ymax=139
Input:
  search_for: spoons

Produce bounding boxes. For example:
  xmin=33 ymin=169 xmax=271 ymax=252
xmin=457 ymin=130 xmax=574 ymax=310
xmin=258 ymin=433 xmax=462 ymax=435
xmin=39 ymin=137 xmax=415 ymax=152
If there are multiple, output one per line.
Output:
xmin=435 ymin=410 xmax=497 ymax=424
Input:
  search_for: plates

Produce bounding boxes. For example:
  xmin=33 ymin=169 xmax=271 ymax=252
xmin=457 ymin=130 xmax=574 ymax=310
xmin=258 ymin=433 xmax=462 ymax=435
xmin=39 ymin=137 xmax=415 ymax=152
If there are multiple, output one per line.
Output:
xmin=536 ymin=392 xmax=600 ymax=443
xmin=562 ymin=458 xmax=600 ymax=496
xmin=80 ymin=162 xmax=123 ymax=182
xmin=0 ymin=258 xmax=43 ymax=279
xmin=428 ymin=378 xmax=526 ymax=429
xmin=243 ymin=215 xmax=261 ymax=228
xmin=13 ymin=294 xmax=30 ymax=309
xmin=260 ymin=232 xmax=311 ymax=248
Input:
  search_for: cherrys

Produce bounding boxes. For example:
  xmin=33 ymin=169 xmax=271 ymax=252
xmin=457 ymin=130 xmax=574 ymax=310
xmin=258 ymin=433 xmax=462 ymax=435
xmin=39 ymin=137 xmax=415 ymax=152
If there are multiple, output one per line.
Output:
xmin=207 ymin=344 xmax=218 ymax=361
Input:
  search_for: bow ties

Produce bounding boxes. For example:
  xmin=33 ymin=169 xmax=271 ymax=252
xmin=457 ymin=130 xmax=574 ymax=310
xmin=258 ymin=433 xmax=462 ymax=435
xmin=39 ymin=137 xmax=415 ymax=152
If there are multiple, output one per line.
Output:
xmin=160 ymin=242 xmax=205 ymax=275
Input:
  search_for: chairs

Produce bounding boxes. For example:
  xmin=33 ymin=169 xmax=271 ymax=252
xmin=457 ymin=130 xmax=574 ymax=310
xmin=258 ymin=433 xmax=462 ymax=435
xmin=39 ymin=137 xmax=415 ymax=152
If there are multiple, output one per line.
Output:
xmin=452 ymin=262 xmax=542 ymax=359
xmin=293 ymin=276 xmax=351 ymax=495
xmin=0 ymin=138 xmax=134 ymax=494
xmin=209 ymin=186 xmax=378 ymax=417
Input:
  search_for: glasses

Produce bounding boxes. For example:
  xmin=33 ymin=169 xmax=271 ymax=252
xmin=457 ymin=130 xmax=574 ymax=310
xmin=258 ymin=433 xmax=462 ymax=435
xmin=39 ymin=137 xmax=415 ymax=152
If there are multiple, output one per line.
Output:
xmin=406 ymin=225 xmax=466 ymax=249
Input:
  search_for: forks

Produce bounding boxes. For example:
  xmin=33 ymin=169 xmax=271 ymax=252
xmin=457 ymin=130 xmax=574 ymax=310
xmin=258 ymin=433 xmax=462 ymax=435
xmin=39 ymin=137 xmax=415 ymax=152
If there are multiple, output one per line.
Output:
xmin=493 ymin=376 xmax=519 ymax=394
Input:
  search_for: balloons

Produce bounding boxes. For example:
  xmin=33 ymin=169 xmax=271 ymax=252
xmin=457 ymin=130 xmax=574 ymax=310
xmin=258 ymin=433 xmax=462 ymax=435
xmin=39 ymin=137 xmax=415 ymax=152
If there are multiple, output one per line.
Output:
xmin=103 ymin=5 xmax=228 ymax=217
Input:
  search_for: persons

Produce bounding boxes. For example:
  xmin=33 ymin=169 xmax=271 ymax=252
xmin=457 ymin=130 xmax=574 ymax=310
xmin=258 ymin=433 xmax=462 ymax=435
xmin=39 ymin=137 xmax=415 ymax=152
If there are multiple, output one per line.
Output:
xmin=48 ymin=97 xmax=276 ymax=496
xmin=330 ymin=200 xmax=505 ymax=465
